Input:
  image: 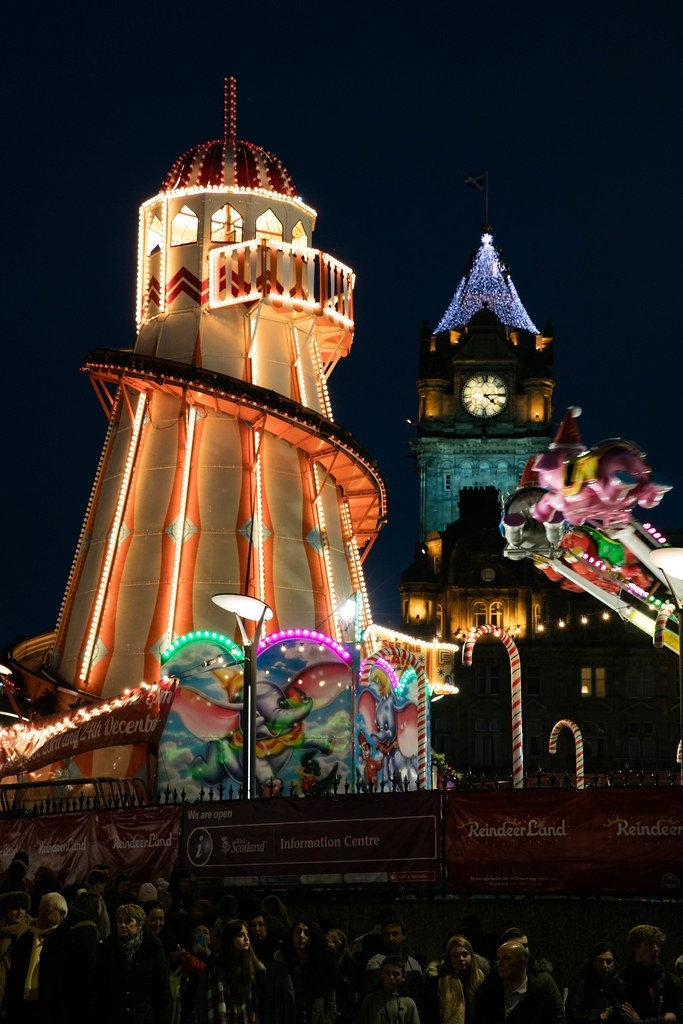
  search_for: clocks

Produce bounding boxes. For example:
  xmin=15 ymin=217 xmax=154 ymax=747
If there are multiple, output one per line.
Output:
xmin=461 ymin=371 xmax=509 ymax=418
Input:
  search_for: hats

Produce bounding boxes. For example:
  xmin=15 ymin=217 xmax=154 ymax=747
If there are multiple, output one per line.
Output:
xmin=0 ymin=891 xmax=32 ymax=910
xmin=138 ymin=883 xmax=158 ymax=902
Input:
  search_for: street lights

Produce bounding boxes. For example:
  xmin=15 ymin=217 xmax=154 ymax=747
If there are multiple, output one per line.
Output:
xmin=209 ymin=591 xmax=275 ymax=802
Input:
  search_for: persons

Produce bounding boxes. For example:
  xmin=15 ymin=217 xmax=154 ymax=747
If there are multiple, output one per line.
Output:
xmin=0 ymin=851 xmax=683 ymax=1024
